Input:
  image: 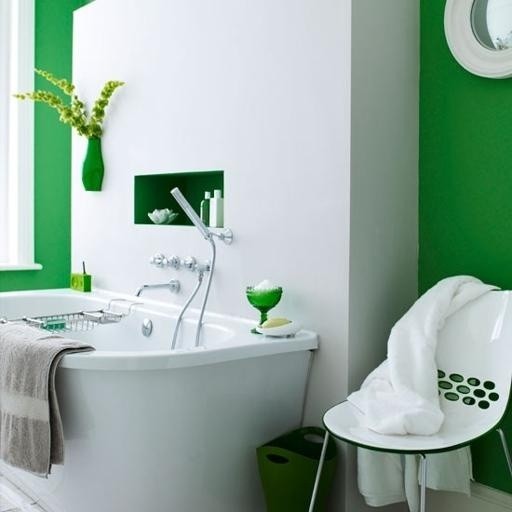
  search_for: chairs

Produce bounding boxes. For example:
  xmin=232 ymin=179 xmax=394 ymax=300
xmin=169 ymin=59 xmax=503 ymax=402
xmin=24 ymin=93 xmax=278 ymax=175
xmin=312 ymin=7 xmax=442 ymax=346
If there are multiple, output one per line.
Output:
xmin=308 ymin=288 xmax=511 ymax=510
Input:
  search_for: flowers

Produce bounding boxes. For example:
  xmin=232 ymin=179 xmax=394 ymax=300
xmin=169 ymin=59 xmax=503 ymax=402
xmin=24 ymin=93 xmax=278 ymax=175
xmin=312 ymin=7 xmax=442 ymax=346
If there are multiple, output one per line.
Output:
xmin=12 ymin=65 xmax=131 ymax=139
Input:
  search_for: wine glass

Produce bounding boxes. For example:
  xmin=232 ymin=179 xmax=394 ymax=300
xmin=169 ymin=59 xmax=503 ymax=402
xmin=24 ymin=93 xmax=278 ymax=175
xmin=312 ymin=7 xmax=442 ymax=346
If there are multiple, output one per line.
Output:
xmin=246 ymin=285 xmax=282 ymax=335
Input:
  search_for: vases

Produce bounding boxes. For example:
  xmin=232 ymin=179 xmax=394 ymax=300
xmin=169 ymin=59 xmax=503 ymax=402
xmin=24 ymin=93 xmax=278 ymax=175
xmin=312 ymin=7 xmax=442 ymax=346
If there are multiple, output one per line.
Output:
xmin=81 ymin=136 xmax=106 ymax=192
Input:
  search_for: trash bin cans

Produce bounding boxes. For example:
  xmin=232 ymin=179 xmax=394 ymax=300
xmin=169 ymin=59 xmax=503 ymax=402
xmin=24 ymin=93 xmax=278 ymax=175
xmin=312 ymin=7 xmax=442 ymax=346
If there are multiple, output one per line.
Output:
xmin=256 ymin=425 xmax=338 ymax=512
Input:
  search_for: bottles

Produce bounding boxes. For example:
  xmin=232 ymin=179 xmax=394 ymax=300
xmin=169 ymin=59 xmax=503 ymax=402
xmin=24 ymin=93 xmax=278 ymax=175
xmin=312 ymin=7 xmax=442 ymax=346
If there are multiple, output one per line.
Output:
xmin=200 ymin=191 xmax=210 ymax=227
xmin=211 ymin=189 xmax=224 ymax=227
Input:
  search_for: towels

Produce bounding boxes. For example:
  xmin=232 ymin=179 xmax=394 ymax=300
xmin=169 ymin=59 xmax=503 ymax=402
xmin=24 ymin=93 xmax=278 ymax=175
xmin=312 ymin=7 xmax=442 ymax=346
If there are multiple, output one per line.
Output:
xmin=347 ymin=273 xmax=503 ymax=511
xmin=0 ymin=322 xmax=96 ymax=479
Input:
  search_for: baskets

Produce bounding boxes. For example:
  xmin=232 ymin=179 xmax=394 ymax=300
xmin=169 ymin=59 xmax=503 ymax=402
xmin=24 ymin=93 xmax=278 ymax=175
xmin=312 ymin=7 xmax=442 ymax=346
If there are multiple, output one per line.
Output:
xmin=26 ymin=312 xmax=100 ymax=334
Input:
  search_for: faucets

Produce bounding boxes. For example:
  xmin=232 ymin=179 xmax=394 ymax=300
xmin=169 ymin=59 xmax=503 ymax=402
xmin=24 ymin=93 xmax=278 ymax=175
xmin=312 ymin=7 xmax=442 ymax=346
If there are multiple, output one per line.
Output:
xmin=134 ymin=279 xmax=180 ymax=297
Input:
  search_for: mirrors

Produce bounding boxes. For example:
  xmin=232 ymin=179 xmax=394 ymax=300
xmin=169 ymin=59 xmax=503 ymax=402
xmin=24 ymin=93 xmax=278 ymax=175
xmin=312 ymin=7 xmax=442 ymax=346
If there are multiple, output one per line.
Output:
xmin=442 ymin=0 xmax=511 ymax=81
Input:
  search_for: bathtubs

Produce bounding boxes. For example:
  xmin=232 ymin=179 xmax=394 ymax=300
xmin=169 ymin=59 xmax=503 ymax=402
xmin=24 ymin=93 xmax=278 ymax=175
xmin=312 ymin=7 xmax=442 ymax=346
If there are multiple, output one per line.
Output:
xmin=0 ymin=286 xmax=319 ymax=512
